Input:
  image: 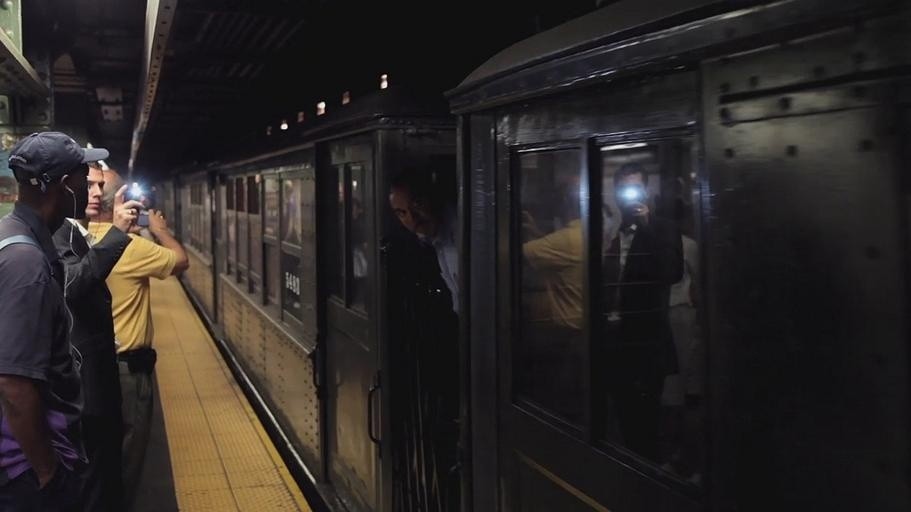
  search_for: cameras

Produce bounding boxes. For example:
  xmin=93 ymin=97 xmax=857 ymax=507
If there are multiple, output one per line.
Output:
xmin=135 ymin=213 xmax=149 ymax=227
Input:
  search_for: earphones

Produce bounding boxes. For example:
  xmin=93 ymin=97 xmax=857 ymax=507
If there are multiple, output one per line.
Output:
xmin=64 ymin=183 xmax=74 ymax=195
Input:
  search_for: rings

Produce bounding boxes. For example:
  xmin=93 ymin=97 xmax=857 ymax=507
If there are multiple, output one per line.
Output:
xmin=637 ymin=208 xmax=641 ymax=213
xmin=129 ymin=208 xmax=134 ymax=215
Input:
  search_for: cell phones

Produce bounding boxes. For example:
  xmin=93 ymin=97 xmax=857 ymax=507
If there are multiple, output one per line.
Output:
xmin=122 ymin=188 xmax=140 ymax=203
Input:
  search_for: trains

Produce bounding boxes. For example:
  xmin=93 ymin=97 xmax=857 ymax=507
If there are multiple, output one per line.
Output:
xmin=149 ymin=1 xmax=909 ymax=508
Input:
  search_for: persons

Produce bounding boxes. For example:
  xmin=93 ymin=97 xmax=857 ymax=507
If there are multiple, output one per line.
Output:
xmin=669 ymin=200 xmax=701 ymax=400
xmin=387 ymin=171 xmax=462 ymax=317
xmin=1 ymin=131 xmax=111 ymax=511
xmin=60 ymin=162 xmax=147 ymax=510
xmin=520 ymin=165 xmax=583 ymax=391
xmin=102 ymin=171 xmax=189 ymax=502
xmin=281 ymin=180 xmax=298 ymax=244
xmin=602 ymin=163 xmax=682 ymax=449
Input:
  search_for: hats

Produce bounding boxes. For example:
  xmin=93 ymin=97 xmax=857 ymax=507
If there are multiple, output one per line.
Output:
xmin=7 ymin=128 xmax=112 ymax=189
